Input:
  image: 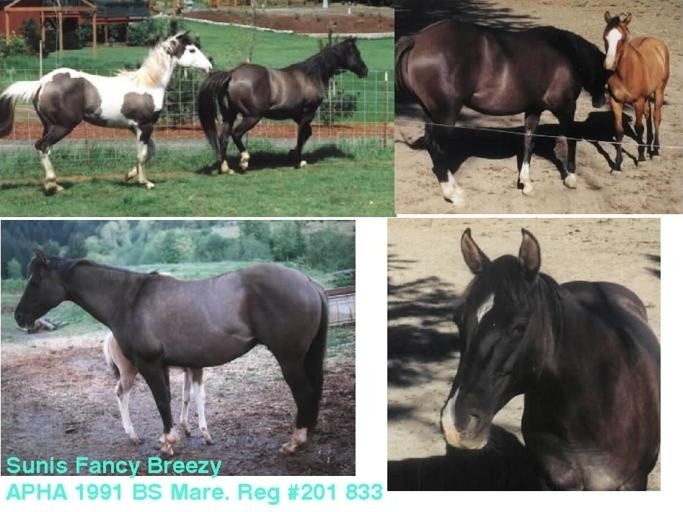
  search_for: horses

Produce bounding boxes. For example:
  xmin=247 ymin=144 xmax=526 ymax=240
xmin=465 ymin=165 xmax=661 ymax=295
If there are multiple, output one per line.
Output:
xmin=12 ymin=245 xmax=330 ymax=458
xmin=440 ymin=226 xmax=660 ymax=491
xmin=601 ymin=9 xmax=670 ymax=175
xmin=99 ymin=324 xmax=214 ymax=448
xmin=0 ymin=26 xmax=215 ymax=199
xmin=192 ymin=35 xmax=370 ymax=176
xmin=394 ymin=15 xmax=612 ymax=207
